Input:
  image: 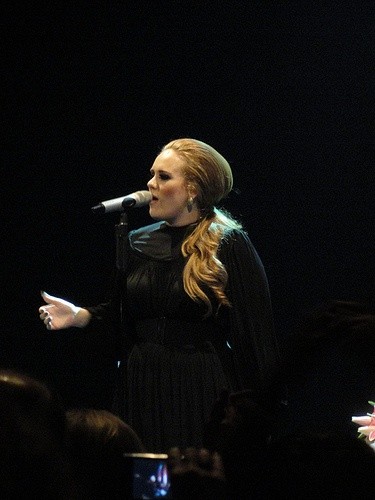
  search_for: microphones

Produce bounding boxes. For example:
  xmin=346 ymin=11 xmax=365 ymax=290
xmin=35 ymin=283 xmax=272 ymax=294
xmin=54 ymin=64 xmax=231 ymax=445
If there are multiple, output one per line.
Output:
xmin=91 ymin=191 xmax=153 ymax=215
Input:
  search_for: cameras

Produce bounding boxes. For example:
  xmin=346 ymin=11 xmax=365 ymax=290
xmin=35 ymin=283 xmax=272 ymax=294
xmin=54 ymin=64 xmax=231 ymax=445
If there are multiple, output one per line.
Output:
xmin=126 ymin=451 xmax=171 ymax=500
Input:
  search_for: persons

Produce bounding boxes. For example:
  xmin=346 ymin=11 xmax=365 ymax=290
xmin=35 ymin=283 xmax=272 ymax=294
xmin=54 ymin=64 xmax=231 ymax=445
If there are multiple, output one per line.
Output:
xmin=1 ymin=298 xmax=375 ymax=500
xmin=39 ymin=138 xmax=289 ymax=448
